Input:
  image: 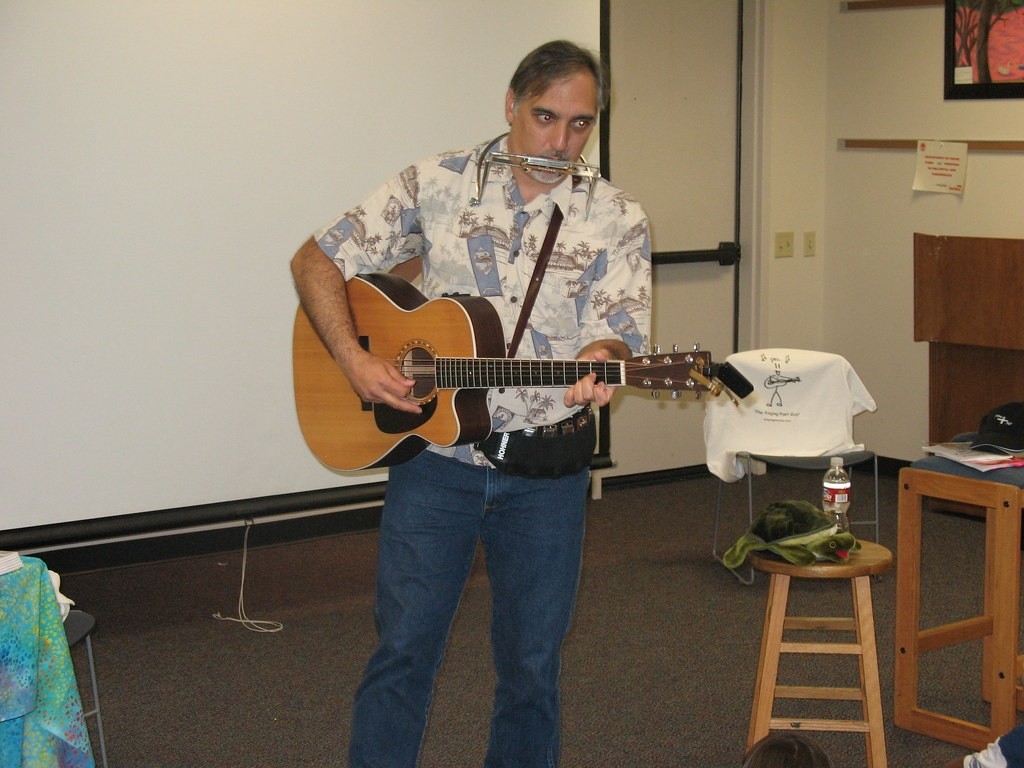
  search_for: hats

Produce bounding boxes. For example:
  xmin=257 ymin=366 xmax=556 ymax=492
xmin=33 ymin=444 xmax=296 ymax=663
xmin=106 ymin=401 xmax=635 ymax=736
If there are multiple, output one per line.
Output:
xmin=970 ymin=402 xmax=1024 ymax=452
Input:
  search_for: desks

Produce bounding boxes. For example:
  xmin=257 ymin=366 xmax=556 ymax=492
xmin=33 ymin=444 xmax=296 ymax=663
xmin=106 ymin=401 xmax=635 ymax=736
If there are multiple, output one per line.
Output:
xmin=0 ymin=555 xmax=96 ymax=768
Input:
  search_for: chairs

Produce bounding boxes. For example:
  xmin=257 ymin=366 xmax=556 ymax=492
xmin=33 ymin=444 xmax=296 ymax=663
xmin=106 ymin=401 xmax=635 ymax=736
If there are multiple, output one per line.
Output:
xmin=61 ymin=611 xmax=109 ymax=768
xmin=712 ymin=346 xmax=879 ymax=584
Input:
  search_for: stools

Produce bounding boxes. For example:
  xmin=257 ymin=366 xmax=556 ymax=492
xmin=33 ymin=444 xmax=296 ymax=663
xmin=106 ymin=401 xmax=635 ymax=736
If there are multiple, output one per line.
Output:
xmin=895 ymin=454 xmax=1024 ymax=751
xmin=744 ymin=537 xmax=892 ymax=768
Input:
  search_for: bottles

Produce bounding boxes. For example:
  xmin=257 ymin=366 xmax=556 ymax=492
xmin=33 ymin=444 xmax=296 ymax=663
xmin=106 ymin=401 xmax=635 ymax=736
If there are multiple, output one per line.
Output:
xmin=822 ymin=456 xmax=851 ymax=535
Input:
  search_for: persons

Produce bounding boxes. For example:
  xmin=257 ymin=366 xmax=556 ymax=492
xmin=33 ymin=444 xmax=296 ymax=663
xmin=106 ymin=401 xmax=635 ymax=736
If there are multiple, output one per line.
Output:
xmin=287 ymin=36 xmax=654 ymax=768
xmin=941 ymin=724 xmax=1024 ymax=768
xmin=740 ymin=732 xmax=830 ymax=768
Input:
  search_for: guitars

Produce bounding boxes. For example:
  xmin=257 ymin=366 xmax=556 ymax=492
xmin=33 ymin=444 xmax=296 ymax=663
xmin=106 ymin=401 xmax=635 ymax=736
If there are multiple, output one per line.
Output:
xmin=290 ymin=271 xmax=758 ymax=479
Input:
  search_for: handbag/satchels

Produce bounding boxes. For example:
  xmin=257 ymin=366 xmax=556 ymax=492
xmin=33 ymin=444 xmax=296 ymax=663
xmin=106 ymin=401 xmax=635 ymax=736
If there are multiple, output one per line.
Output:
xmin=474 ymin=403 xmax=596 ymax=479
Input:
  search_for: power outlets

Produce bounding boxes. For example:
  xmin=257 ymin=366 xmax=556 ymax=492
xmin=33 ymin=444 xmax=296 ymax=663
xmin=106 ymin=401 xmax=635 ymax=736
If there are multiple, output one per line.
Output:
xmin=804 ymin=231 xmax=816 ymax=257
xmin=775 ymin=232 xmax=793 ymax=258
